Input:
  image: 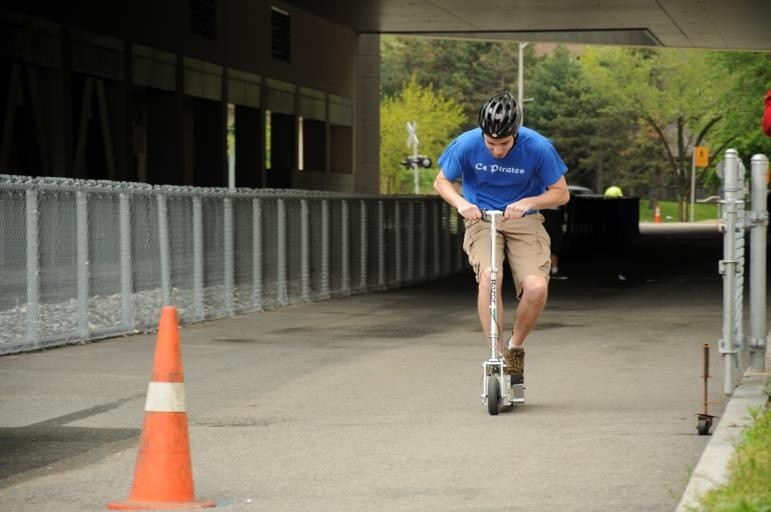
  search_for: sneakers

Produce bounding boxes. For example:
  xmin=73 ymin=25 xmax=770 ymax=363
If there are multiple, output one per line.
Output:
xmin=485 ymin=365 xmax=499 ymax=376
xmin=503 ymin=333 xmax=525 ymax=385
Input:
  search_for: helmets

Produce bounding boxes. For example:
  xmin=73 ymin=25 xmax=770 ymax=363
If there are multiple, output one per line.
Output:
xmin=478 ymin=91 xmax=522 ymax=139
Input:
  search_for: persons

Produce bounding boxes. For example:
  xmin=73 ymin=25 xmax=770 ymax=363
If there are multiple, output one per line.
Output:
xmin=433 ymin=90 xmax=570 ymax=385
xmin=539 ymin=208 xmax=568 ymax=280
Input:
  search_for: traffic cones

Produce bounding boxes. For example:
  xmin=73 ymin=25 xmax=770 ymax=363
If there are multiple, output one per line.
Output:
xmin=104 ymin=302 xmax=217 ymax=511
xmin=653 ymin=203 xmax=664 ymax=224
